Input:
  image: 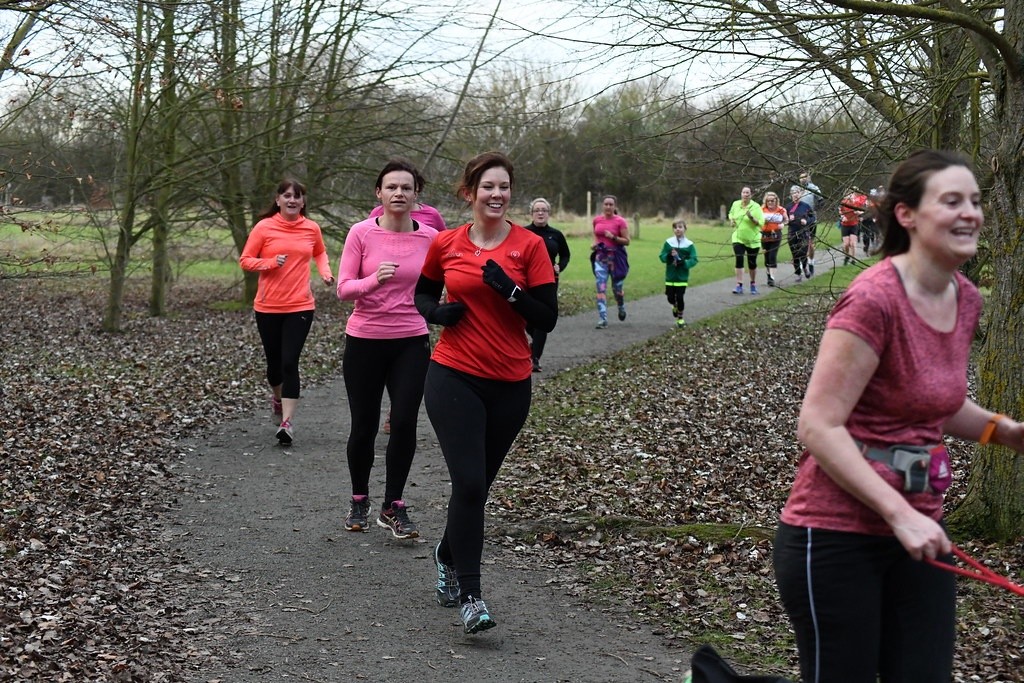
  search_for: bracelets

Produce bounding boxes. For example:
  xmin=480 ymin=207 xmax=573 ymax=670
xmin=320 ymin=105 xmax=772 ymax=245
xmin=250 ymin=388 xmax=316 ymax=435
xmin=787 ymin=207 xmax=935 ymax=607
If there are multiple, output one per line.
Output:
xmin=612 ymin=235 xmax=617 ymax=241
xmin=749 ymin=217 xmax=753 ymax=220
xmin=978 ymin=413 xmax=1007 ymax=449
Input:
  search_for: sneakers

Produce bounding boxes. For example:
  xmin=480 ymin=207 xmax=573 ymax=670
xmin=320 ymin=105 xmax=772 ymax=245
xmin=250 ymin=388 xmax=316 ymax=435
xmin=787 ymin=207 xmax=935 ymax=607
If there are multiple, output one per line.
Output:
xmin=751 ymin=283 xmax=757 ymax=294
xmin=433 ymin=540 xmax=461 ymax=606
xmin=275 ymin=418 xmax=294 ymax=444
xmin=377 ymin=499 xmax=420 ymax=539
xmin=596 ymin=317 xmax=608 ymax=328
xmin=344 ymin=493 xmax=371 ymax=531
xmin=271 ymin=397 xmax=283 ymax=425
xmin=532 ymin=356 xmax=542 ymax=372
xmin=732 ymin=283 xmax=744 ymax=294
xmin=676 ymin=318 xmax=687 ymax=328
xmin=618 ymin=305 xmax=626 ymax=321
xmin=673 ymin=304 xmax=679 ymax=318
xmin=459 ymin=594 xmax=497 ymax=633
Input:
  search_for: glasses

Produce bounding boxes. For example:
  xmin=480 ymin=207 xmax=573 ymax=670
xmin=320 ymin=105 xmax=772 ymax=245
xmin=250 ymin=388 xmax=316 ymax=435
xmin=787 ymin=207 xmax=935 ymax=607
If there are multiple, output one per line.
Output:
xmin=532 ymin=208 xmax=550 ymax=213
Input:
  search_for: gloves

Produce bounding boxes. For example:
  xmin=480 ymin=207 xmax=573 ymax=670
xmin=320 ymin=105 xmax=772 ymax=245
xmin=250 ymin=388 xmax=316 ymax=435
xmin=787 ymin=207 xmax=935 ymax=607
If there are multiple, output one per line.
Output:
xmin=479 ymin=258 xmax=522 ymax=303
xmin=431 ymin=301 xmax=469 ymax=327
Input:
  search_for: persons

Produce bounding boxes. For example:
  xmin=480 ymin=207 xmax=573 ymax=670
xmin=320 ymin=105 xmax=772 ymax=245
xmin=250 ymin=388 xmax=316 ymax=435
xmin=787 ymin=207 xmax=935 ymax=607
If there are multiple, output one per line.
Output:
xmin=786 ymin=184 xmax=816 ymax=282
xmin=411 ymin=152 xmax=559 ymax=636
xmin=679 ymin=143 xmax=1023 ymax=683
xmin=240 ymin=179 xmax=337 ymax=450
xmin=760 ymin=191 xmax=789 ymax=287
xmin=331 ymin=157 xmax=447 ymax=539
xmin=588 ymin=194 xmax=631 ymax=330
xmin=797 ymin=171 xmax=823 ymax=276
xmin=728 ymin=185 xmax=765 ymax=295
xmin=659 ymin=218 xmax=698 ymax=327
xmin=364 ymin=159 xmax=447 ymax=435
xmin=515 ymin=196 xmax=570 ymax=374
xmin=839 ymin=185 xmax=887 ymax=265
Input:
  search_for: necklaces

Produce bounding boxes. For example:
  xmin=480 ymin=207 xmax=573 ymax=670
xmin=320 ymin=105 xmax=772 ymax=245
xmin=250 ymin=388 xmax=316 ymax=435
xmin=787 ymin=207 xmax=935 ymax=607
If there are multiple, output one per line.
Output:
xmin=472 ymin=222 xmax=506 ymax=256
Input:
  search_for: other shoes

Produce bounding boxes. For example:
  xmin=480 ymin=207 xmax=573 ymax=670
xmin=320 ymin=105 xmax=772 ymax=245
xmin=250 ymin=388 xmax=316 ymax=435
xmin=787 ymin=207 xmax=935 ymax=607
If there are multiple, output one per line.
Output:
xmin=795 ymin=273 xmax=801 ymax=283
xmin=802 ymin=265 xmax=810 ymax=278
xmin=844 ymin=256 xmax=849 ymax=266
xmin=851 ymin=259 xmax=856 ymax=265
xmin=808 ymin=259 xmax=815 ymax=274
xmin=384 ymin=405 xmax=391 ymax=434
xmin=767 ymin=276 xmax=776 ymax=286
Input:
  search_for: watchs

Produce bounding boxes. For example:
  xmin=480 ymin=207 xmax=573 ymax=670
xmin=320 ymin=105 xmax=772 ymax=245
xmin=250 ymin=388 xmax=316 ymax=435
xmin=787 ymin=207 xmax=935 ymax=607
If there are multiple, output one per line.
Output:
xmin=507 ymin=287 xmax=521 ymax=303
